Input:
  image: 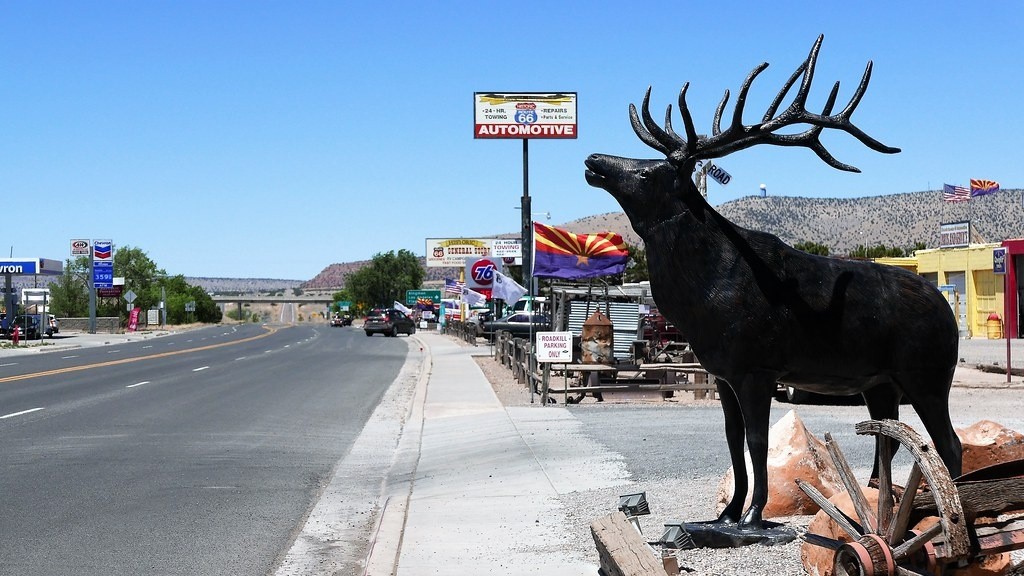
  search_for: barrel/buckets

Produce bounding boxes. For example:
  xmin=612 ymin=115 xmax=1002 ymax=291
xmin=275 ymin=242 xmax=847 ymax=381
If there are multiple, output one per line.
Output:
xmin=987 ymin=319 xmax=1001 ymax=339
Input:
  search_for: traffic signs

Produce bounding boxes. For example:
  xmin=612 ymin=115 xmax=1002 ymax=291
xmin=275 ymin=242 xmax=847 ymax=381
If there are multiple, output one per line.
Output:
xmin=93 ymin=240 xmax=111 ymax=289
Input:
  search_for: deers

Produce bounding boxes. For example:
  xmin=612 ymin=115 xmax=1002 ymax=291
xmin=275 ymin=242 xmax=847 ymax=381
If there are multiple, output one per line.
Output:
xmin=584 ymin=32 xmax=963 ymax=531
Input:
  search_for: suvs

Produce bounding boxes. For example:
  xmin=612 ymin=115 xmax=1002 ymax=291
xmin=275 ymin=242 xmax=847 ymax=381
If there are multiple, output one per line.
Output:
xmin=364 ymin=308 xmax=415 ymax=337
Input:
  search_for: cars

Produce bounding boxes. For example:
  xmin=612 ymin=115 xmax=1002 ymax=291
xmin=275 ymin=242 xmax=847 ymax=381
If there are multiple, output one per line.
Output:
xmin=330 ymin=312 xmax=351 ymax=327
xmin=465 ymin=297 xmax=551 ymax=345
xmin=0 ymin=313 xmax=59 ymax=340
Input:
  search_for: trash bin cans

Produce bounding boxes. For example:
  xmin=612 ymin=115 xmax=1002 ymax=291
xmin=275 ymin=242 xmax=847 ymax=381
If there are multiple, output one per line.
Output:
xmin=148 ymin=310 xmax=159 ymax=324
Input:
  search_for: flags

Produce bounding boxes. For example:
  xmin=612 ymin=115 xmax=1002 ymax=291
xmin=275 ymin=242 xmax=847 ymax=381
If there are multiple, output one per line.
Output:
xmin=394 ymin=301 xmax=412 ymax=316
xmin=446 ymin=278 xmax=465 ymax=294
xmin=490 ymin=270 xmax=528 ymax=306
xmin=943 ymin=184 xmax=972 ymax=201
xmin=531 ymin=221 xmax=628 ymax=280
xmin=971 ymin=178 xmax=999 ymax=197
xmin=462 ymin=288 xmax=487 ymax=307
xmin=417 ymin=298 xmax=434 ymax=312
